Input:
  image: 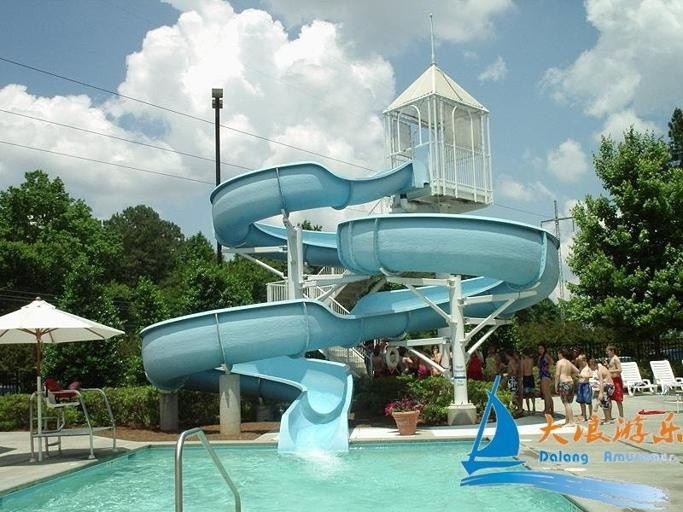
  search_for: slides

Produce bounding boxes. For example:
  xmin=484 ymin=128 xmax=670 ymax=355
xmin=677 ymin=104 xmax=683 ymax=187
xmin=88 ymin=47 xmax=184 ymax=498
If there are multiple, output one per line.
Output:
xmin=139 ymin=162 xmax=559 ymax=456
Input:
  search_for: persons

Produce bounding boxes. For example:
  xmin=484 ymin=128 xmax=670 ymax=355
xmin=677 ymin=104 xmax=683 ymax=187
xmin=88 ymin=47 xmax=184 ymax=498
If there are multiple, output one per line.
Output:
xmin=364 ymin=335 xmax=625 ymax=428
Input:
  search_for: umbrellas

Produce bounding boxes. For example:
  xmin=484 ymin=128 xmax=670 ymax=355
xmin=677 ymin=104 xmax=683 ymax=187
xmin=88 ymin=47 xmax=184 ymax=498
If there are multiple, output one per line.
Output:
xmin=0 ymin=296 xmax=124 ymax=463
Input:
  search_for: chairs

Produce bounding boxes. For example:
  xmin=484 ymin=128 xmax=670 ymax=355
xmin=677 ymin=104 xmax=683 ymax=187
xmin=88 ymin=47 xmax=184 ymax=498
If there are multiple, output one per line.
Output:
xmin=621 ymin=360 xmax=683 ymax=397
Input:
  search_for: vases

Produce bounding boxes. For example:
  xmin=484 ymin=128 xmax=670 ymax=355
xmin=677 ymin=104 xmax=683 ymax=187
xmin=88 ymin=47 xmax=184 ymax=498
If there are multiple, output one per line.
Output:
xmin=392 ymin=409 xmax=419 ymax=435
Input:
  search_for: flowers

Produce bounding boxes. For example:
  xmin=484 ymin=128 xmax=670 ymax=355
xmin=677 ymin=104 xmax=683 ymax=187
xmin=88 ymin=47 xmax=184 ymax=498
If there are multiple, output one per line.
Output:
xmin=383 ymin=398 xmax=426 ymax=415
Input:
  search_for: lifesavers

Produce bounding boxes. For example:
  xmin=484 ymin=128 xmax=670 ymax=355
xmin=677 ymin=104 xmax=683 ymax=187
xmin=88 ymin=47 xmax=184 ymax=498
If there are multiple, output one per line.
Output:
xmin=386 ymin=348 xmax=400 ymax=370
